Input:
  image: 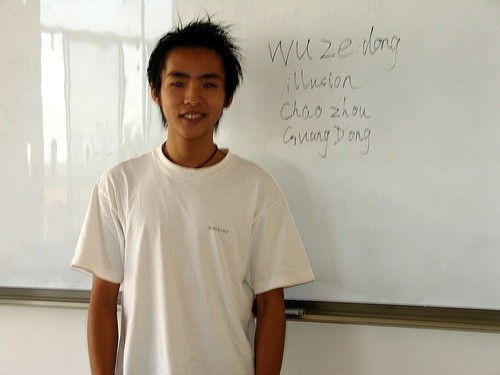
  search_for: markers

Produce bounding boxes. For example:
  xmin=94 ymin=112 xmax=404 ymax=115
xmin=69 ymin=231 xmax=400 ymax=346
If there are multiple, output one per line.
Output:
xmin=284 ymin=307 xmax=305 ymax=319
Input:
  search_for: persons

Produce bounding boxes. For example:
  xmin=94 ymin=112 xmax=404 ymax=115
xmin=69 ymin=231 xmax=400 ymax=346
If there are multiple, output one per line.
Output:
xmin=71 ymin=8 xmax=314 ymax=375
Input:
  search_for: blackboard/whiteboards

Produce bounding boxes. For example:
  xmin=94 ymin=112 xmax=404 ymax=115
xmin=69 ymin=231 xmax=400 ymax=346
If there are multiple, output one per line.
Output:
xmin=1 ymin=0 xmax=500 ymax=333
